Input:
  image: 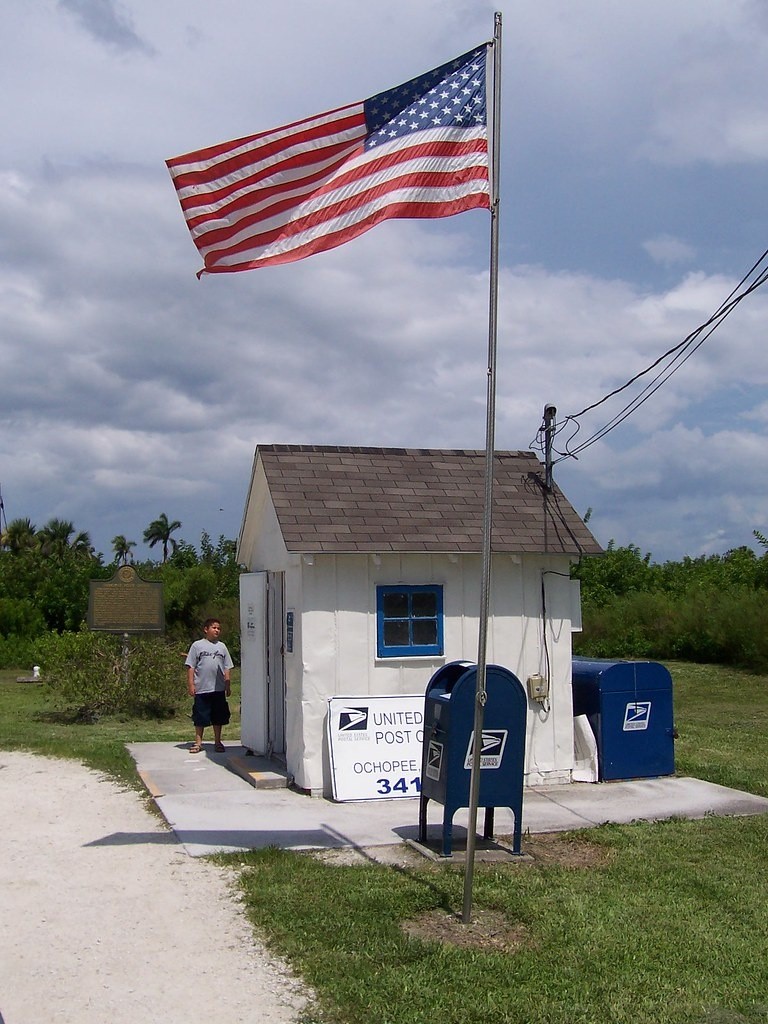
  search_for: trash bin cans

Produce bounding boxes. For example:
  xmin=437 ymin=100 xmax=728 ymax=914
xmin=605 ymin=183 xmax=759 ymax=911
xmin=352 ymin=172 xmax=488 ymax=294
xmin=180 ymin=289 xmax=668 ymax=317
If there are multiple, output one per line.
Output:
xmin=421 ymin=660 xmax=527 ymax=808
xmin=573 ymin=659 xmax=675 ymax=777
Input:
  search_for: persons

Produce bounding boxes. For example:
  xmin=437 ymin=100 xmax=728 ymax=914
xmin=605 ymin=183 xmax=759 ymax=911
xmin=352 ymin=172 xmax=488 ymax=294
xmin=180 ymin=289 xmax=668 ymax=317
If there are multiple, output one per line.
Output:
xmin=185 ymin=618 xmax=234 ymax=753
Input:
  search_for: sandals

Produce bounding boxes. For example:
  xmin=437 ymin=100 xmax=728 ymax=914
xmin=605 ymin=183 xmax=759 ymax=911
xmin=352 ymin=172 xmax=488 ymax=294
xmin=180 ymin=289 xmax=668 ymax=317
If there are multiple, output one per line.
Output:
xmin=215 ymin=742 xmax=224 ymax=752
xmin=189 ymin=743 xmax=202 ymax=753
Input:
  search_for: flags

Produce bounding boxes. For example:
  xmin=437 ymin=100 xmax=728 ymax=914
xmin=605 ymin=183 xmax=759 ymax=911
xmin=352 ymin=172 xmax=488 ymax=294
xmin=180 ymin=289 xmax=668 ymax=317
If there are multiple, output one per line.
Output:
xmin=163 ymin=37 xmax=502 ymax=280
